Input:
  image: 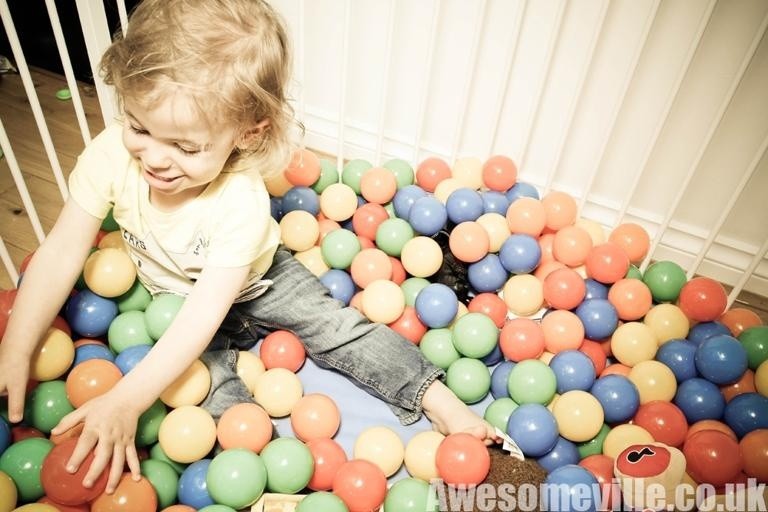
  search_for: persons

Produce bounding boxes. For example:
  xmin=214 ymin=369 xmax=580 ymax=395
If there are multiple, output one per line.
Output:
xmin=1 ymin=1 xmax=504 ymax=501
xmin=0 ymin=143 xmax=767 ymax=511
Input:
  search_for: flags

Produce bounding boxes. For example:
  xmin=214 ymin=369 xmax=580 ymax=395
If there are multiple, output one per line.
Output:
xmin=0 ymin=143 xmax=767 ymax=511
